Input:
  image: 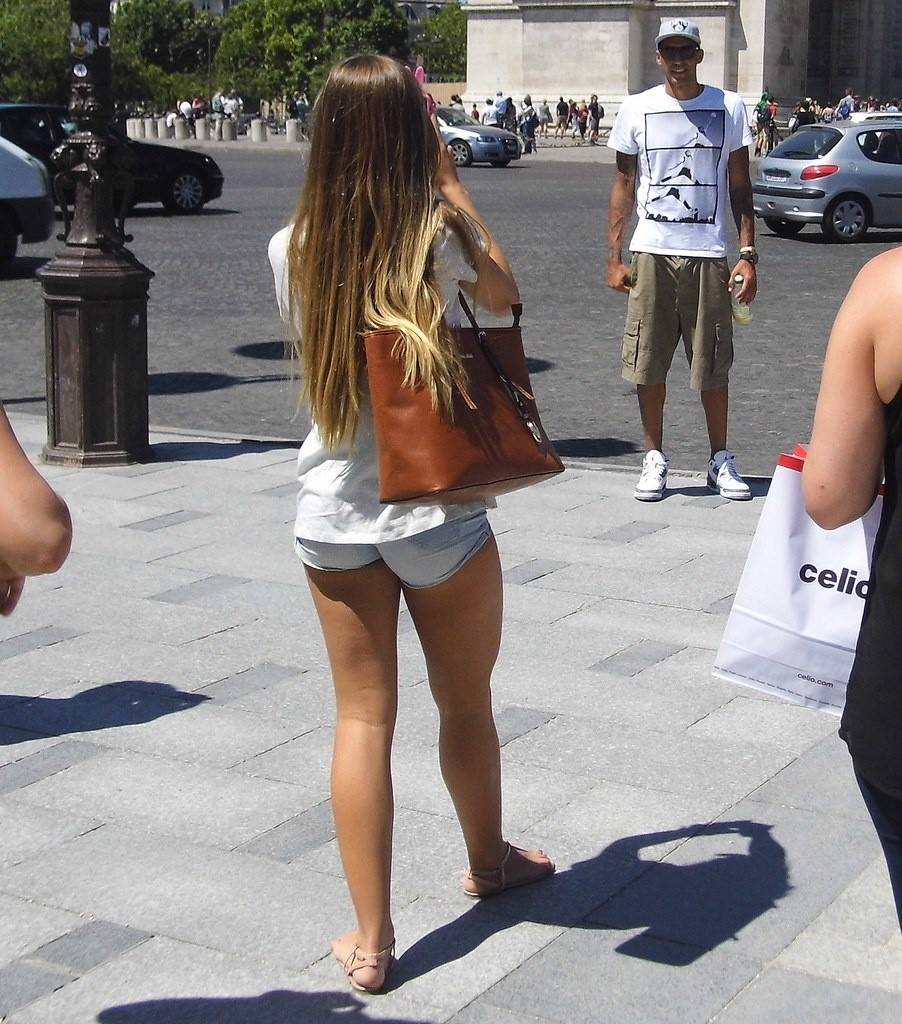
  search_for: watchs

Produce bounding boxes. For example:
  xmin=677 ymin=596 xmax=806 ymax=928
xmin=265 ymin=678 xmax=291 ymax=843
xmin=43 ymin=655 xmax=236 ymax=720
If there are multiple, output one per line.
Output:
xmin=739 ymin=253 xmax=758 ymax=265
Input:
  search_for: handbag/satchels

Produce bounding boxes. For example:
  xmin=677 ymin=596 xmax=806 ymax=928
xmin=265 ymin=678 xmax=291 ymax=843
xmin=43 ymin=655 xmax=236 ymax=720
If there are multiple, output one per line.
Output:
xmin=366 ymin=198 xmax=566 ymax=504
xmin=525 ymin=112 xmax=538 ymax=130
xmin=788 ymin=112 xmax=799 ymax=133
xmin=715 ymin=441 xmax=885 ymax=713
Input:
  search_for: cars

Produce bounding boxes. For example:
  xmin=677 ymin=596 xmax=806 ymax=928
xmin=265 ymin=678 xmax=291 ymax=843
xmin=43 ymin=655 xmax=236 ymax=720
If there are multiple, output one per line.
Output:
xmin=846 ymin=112 xmax=902 ymax=146
xmin=436 ymin=106 xmax=522 ymax=168
xmin=752 ymin=118 xmax=902 ymax=242
xmin=0 ymin=104 xmax=225 ymax=215
xmin=1 ymin=137 xmax=55 ymax=268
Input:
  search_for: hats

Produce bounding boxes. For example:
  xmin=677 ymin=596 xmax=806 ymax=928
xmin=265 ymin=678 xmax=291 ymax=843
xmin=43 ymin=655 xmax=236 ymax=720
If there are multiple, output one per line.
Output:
xmin=655 ymin=21 xmax=700 ymax=44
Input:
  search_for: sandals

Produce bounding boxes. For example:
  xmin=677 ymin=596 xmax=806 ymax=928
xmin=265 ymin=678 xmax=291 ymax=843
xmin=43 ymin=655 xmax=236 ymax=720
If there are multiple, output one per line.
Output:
xmin=463 ymin=842 xmax=554 ymax=898
xmin=337 ymin=936 xmax=398 ymax=991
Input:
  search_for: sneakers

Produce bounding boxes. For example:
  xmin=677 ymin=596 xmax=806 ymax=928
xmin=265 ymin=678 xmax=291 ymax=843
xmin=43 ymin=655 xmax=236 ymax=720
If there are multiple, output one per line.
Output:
xmin=708 ymin=448 xmax=752 ymax=500
xmin=635 ymin=449 xmax=667 ymax=501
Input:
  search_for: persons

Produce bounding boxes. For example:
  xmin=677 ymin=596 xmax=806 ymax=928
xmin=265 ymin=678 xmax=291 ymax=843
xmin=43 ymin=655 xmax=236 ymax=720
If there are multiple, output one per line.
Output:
xmin=752 ymin=87 xmax=902 ymax=157
xmin=0 ymin=398 xmax=72 ymax=616
xmin=176 ymin=89 xmax=309 ymax=136
xmin=606 ymin=18 xmax=759 ymax=501
xmin=449 ymin=91 xmax=605 ymax=154
xmin=268 ymin=53 xmax=555 ymax=996
xmin=800 ymin=246 xmax=902 ymax=931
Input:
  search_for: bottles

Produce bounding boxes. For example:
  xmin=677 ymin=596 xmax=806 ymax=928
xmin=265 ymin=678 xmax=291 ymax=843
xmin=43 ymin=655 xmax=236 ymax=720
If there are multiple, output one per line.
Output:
xmin=730 ymin=275 xmax=753 ymax=324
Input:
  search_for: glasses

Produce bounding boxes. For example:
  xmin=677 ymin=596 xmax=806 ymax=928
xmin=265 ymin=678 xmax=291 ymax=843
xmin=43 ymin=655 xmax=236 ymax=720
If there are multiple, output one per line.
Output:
xmin=658 ymin=45 xmax=699 ymax=62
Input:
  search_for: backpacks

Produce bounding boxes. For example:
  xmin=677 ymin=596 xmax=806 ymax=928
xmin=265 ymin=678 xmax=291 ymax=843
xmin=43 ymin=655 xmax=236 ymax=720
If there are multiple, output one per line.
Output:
xmin=836 ymin=101 xmax=850 ymax=121
xmin=757 ymin=107 xmax=771 ymax=123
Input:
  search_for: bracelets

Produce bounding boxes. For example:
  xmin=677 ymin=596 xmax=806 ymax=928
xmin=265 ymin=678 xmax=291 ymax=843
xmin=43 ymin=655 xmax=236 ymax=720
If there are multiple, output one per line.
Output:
xmin=740 ymin=246 xmax=756 ymax=254
